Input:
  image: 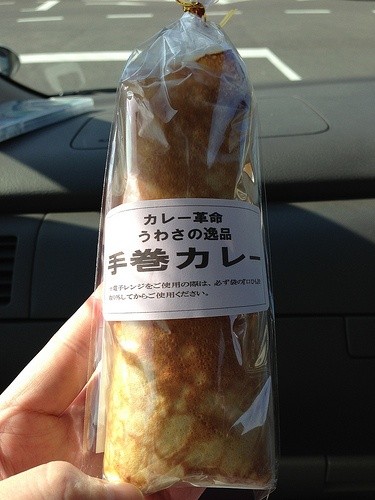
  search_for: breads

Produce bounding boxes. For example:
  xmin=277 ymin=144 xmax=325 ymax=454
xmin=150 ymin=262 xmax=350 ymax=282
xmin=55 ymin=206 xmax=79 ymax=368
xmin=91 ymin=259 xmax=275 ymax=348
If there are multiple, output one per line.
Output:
xmin=100 ymin=49 xmax=280 ymax=491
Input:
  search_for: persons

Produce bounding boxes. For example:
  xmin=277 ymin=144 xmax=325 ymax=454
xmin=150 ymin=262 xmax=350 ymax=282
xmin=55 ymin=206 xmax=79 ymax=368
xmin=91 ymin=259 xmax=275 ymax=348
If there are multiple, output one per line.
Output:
xmin=0 ymin=277 xmax=213 ymax=499
